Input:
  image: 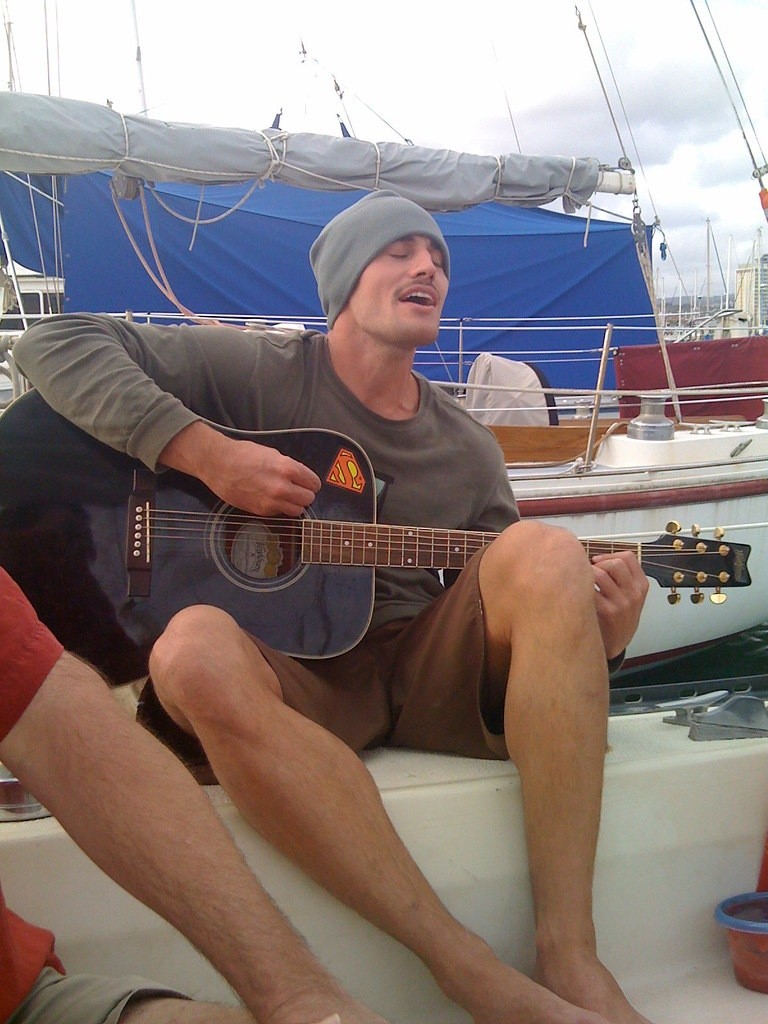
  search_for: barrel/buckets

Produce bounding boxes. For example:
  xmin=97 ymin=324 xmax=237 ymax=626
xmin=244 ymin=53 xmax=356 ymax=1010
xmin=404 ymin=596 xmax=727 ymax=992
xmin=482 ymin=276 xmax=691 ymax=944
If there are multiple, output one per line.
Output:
xmin=714 ymin=891 xmax=768 ymax=994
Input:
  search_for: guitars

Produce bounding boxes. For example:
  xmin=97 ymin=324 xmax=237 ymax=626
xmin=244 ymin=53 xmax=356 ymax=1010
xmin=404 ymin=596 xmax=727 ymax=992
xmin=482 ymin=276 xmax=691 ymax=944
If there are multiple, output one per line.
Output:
xmin=0 ymin=383 xmax=752 ymax=689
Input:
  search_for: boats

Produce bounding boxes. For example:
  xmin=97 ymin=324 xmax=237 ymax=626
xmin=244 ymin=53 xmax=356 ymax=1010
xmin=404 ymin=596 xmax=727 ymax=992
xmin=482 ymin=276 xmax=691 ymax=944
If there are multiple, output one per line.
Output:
xmin=0 ymin=0 xmax=768 ymax=697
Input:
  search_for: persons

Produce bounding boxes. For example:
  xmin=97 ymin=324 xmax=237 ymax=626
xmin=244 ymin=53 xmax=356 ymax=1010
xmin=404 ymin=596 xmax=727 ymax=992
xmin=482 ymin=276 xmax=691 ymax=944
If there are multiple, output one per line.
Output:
xmin=13 ymin=193 xmax=654 ymax=1024
xmin=0 ymin=567 xmax=391 ymax=1024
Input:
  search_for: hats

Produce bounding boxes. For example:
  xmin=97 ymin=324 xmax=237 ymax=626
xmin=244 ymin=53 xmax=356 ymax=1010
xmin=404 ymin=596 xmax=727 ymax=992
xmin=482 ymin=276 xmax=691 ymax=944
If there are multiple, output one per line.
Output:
xmin=309 ymin=190 xmax=451 ymax=330
